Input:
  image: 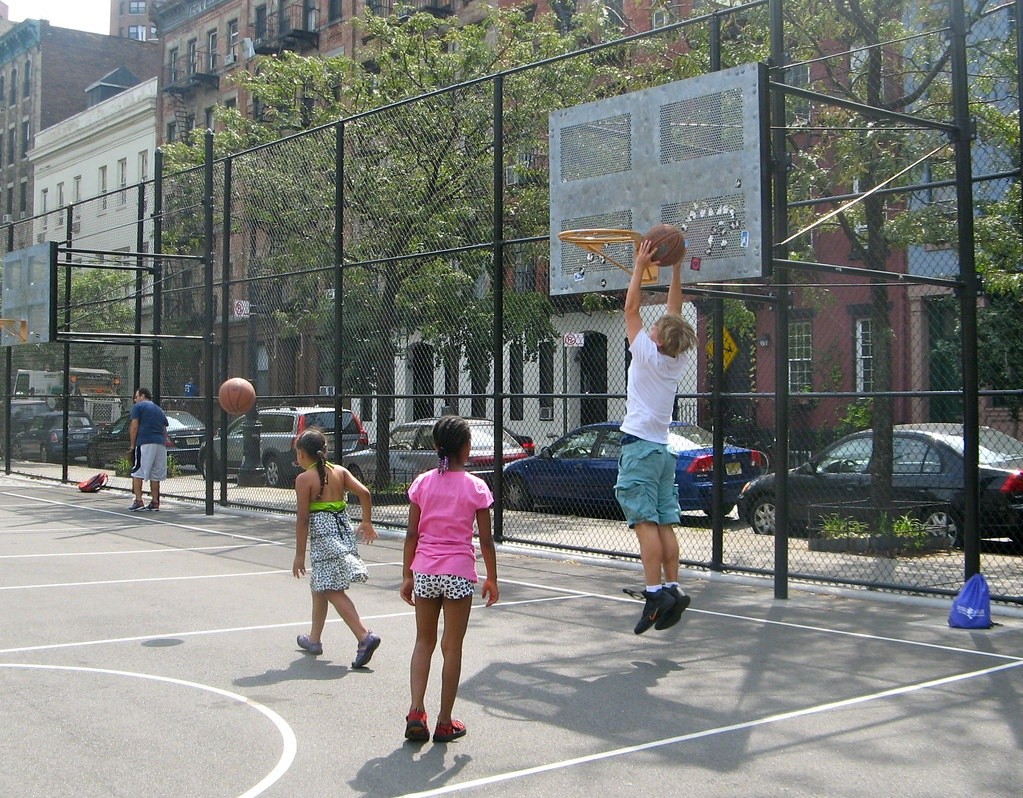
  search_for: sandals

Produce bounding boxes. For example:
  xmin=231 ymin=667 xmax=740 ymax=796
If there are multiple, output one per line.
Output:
xmin=404 ymin=710 xmax=431 ymax=740
xmin=433 ymin=720 xmax=467 ymax=741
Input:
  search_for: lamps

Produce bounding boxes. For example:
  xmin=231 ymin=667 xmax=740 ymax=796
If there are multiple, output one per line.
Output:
xmin=759 ymin=332 xmax=771 ymax=348
xmin=573 ymin=350 xmax=582 ymax=362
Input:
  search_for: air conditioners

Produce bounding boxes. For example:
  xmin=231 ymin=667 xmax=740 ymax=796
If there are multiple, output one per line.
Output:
xmin=321 ymin=288 xmax=336 ymax=300
xmin=319 ymin=385 xmax=336 ymax=396
xmin=505 ymin=162 xmax=525 ymax=186
xmin=539 ymin=406 xmax=554 ymax=421
xmin=388 ymin=407 xmax=394 ymax=421
xmin=3 ymin=214 xmax=13 ymax=225
xmin=223 ymin=54 xmax=237 ymax=65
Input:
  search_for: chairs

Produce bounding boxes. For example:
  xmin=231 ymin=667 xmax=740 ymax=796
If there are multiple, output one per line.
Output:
xmin=416 ymin=435 xmax=435 ymax=449
xmin=68 ymin=420 xmax=84 ymax=428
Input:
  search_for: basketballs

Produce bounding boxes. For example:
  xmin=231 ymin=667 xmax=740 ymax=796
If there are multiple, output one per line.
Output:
xmin=643 ymin=224 xmax=686 ymax=267
xmin=219 ymin=377 xmax=256 ymax=415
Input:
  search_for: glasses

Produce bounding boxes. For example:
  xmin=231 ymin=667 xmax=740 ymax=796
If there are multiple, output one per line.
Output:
xmin=134 ymin=395 xmax=140 ymax=399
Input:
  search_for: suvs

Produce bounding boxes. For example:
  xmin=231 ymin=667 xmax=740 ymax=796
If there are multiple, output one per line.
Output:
xmin=197 ymin=404 xmax=370 ymax=491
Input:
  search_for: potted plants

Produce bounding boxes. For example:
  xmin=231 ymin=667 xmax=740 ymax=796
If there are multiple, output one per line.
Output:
xmin=798 ymin=386 xmax=812 ymax=405
xmin=866 ymin=397 xmax=875 ymax=411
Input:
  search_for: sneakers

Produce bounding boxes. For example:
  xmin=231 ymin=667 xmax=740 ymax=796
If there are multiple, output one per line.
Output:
xmin=145 ymin=502 xmax=159 ymax=511
xmin=298 ymin=635 xmax=323 ymax=655
xmin=634 ymin=586 xmax=691 ymax=635
xmin=351 ymin=630 xmax=381 ymax=668
xmin=128 ymin=500 xmax=144 ymax=511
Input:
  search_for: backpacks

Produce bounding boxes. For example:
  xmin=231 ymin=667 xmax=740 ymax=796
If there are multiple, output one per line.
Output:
xmin=78 ymin=473 xmax=109 ymax=493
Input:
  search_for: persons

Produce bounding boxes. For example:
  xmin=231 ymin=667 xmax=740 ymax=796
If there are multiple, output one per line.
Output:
xmin=126 ymin=387 xmax=168 ymax=511
xmin=398 ymin=416 xmax=499 ymax=745
xmin=614 ymin=239 xmax=701 ymax=635
xmin=292 ymin=429 xmax=380 ymax=669
xmin=180 ymin=377 xmax=201 ymax=412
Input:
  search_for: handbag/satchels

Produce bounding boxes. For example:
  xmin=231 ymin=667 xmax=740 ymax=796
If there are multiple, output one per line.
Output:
xmin=948 ymin=572 xmax=993 ymax=629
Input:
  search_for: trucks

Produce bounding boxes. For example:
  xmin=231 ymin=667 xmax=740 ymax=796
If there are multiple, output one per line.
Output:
xmin=14 ymin=364 xmax=123 ymax=431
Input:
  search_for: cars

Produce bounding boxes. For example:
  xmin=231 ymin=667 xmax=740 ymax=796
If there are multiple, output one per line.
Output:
xmin=503 ymin=420 xmax=766 ymax=520
xmin=339 ymin=413 xmax=528 ymax=500
xmin=8 ymin=408 xmax=99 ymax=464
xmin=85 ymin=408 xmax=208 ymax=470
xmin=735 ymin=421 xmax=1023 ymax=560
xmin=1 ymin=400 xmax=53 ymax=461
